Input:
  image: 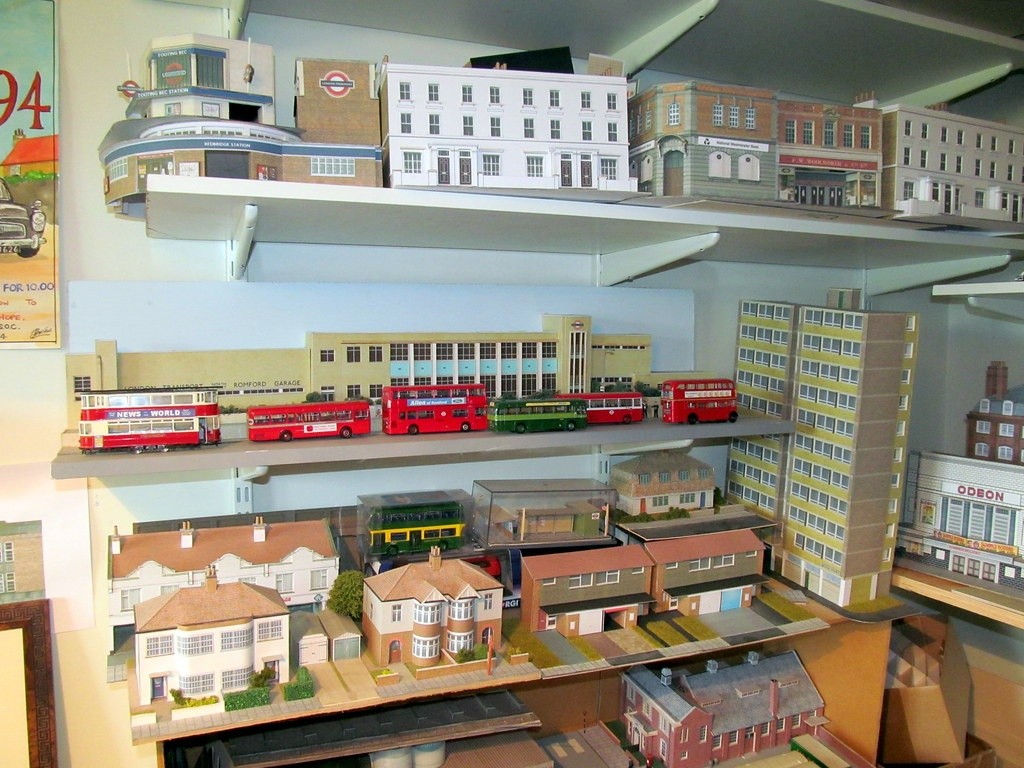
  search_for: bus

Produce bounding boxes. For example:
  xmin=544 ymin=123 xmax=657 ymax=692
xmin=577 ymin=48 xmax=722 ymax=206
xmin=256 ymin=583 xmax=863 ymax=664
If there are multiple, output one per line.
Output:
xmin=487 ymin=397 xmax=588 ymax=434
xmin=365 ymin=503 xmax=466 ymax=555
xmin=78 ymin=392 xmax=222 ymax=452
xmin=660 ymin=378 xmax=739 ymax=423
xmin=381 ymin=384 xmax=490 ymax=435
xmin=247 ymin=400 xmax=370 ymax=443
xmin=553 ymin=392 xmax=643 ymax=424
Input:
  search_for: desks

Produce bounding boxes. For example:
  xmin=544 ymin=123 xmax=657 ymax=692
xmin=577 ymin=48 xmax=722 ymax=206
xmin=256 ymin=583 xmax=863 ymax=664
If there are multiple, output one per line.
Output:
xmin=892 ymin=567 xmax=1024 ymax=768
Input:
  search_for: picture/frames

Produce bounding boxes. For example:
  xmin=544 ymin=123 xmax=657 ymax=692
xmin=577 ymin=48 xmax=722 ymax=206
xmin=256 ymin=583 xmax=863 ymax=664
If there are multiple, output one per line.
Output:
xmin=0 ymin=600 xmax=59 ymax=768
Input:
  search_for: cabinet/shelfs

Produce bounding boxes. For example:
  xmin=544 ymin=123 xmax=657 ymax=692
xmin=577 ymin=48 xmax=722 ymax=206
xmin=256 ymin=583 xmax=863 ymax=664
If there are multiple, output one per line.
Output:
xmin=51 ymin=0 xmax=1024 ymax=768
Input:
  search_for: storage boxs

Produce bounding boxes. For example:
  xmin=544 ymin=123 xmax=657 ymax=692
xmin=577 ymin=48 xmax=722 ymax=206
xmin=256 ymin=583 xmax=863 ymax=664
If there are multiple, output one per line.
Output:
xmin=877 ymin=613 xmax=998 ymax=767
